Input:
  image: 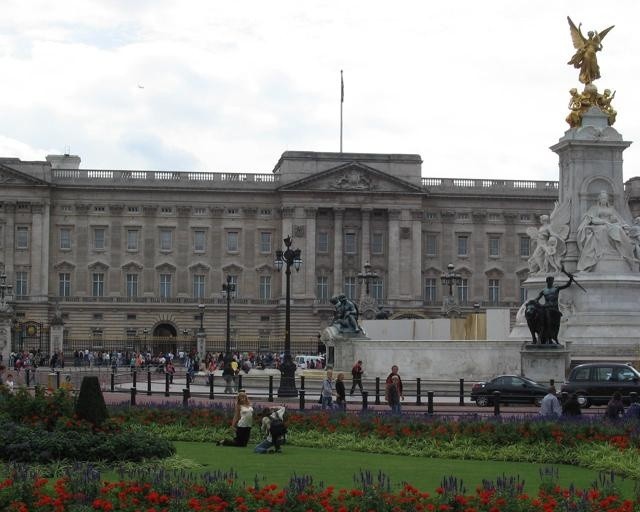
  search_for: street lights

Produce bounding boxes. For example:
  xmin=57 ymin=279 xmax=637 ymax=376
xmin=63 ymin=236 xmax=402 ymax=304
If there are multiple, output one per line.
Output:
xmin=357 ymin=259 xmax=381 ymax=295
xmin=439 ymin=263 xmax=463 ymax=297
xmin=183 ymin=328 xmax=189 ymax=358
xmin=198 ymin=302 xmax=208 ymax=333
xmin=0 ymin=271 xmax=15 ymax=310
xmin=218 ymin=275 xmax=238 ymax=371
xmin=143 ymin=327 xmax=149 ymax=349
xmin=272 ymin=234 xmax=307 ymax=399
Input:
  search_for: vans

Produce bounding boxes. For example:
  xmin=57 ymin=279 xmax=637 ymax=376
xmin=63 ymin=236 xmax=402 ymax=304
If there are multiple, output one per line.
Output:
xmin=294 ymin=355 xmax=326 ymax=368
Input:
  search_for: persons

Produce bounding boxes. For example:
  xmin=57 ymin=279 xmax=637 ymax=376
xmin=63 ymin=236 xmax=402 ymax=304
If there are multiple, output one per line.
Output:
xmin=300 ymin=358 xmax=324 ymax=369
xmin=624 ymin=392 xmax=640 ymax=419
xmin=157 ymin=349 xmax=202 ymax=383
xmin=252 ymin=406 xmax=285 ymax=454
xmin=216 ymin=391 xmax=253 ymax=446
xmin=389 ymin=376 xmax=402 ymax=414
xmin=527 ymin=215 xmax=556 ymax=274
xmin=566 ymin=22 xmax=617 ymax=126
xmin=385 ymin=366 xmax=405 ymax=401
xmin=204 ymin=350 xmax=285 ymax=391
xmin=74 ymin=348 xmax=151 ymax=370
xmin=1 ymin=348 xmax=44 ymax=391
xmin=580 ymin=190 xmax=631 ymax=257
xmin=321 ymin=370 xmax=340 ymax=409
xmin=564 ymin=392 xmax=582 ymax=417
xmin=539 ymin=385 xmax=563 ymax=416
xmin=51 ymin=350 xmax=64 ymax=368
xmin=534 ymin=274 xmax=573 ymax=344
xmin=602 ymin=391 xmax=626 ymax=419
xmin=335 ymin=373 xmax=346 ymax=408
xmin=350 ymin=360 xmax=366 ymax=396
xmin=326 ymin=295 xmax=365 ymax=335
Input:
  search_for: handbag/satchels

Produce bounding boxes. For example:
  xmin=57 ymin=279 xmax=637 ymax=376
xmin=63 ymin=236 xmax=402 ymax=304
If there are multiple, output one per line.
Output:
xmin=318 ymin=394 xmax=322 ymax=404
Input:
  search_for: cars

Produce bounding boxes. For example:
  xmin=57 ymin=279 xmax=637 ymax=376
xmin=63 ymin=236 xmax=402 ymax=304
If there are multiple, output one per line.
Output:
xmin=469 ymin=374 xmax=560 ymax=408
xmin=559 ymin=361 xmax=640 ymax=410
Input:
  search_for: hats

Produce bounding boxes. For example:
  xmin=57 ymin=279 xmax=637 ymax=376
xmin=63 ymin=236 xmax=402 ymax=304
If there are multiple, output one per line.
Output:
xmin=263 ymin=407 xmax=272 ymax=415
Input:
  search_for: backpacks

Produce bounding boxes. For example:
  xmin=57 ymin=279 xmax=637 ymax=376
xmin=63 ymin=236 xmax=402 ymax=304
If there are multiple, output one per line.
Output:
xmin=267 ymin=411 xmax=288 ymax=440
